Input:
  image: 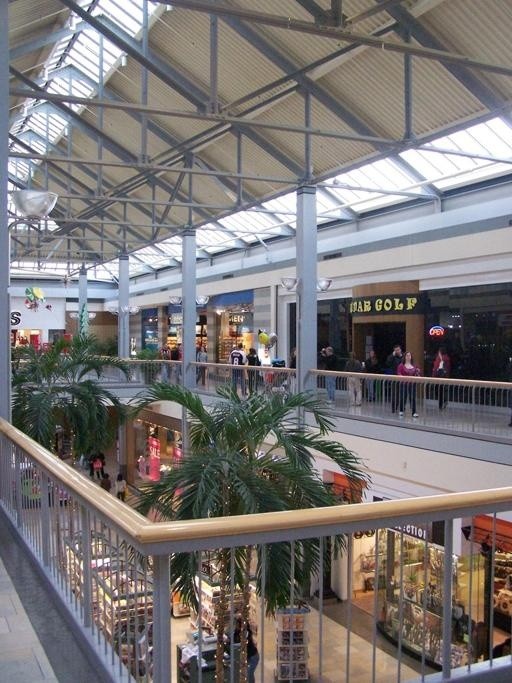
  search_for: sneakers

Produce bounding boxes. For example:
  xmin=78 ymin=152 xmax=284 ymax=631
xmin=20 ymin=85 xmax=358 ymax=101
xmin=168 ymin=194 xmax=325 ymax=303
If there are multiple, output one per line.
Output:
xmin=350 ymin=402 xmax=361 ymax=406
xmin=390 ymin=408 xmax=419 ymax=417
xmin=439 ymin=401 xmax=449 ymax=409
xmin=325 ymin=399 xmax=334 ymax=404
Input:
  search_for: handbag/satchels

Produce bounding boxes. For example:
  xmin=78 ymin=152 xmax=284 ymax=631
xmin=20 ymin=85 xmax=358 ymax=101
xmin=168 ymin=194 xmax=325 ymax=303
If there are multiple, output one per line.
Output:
xmin=123 ymin=480 xmax=128 ymax=500
xmin=435 ymin=354 xmax=448 ymax=378
xmin=414 ymin=366 xmax=420 ymax=376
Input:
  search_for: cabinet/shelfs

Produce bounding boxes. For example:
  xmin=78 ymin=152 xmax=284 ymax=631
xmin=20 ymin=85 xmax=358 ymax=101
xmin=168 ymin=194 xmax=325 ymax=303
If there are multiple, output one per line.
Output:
xmin=361 ymin=539 xmax=423 ymax=599
xmin=190 ymin=580 xmax=250 ymax=633
xmin=64 ymin=536 xmax=154 ymax=665
xmin=219 ymin=338 xmax=236 ymax=360
xmin=273 ymin=605 xmax=310 ymax=682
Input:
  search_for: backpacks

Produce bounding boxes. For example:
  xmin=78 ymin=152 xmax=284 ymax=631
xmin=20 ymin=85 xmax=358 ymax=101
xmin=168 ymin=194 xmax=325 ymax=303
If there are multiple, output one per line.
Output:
xmin=171 ymin=347 xmax=181 ymax=360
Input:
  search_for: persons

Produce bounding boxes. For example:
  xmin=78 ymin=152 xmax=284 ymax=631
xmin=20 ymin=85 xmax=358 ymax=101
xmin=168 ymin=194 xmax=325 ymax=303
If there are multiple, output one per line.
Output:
xmin=90 ymin=451 xmax=126 ymax=501
xmin=320 ymin=343 xmax=451 ymax=417
xmin=234 ymin=618 xmax=259 ymax=683
xmin=161 ymin=343 xmax=296 ymax=395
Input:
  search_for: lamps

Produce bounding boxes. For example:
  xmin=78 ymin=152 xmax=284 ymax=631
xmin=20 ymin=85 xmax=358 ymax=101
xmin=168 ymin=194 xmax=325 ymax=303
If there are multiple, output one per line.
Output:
xmin=129 ymin=306 xmax=141 ymax=316
xmin=88 ymin=312 xmax=97 ymax=321
xmin=283 ymin=276 xmax=302 ymax=293
xmin=195 ymin=294 xmax=210 ymax=309
xmin=69 ymin=312 xmax=80 ymax=321
xmin=5 ymin=186 xmax=60 ymax=232
xmin=106 ymin=303 xmax=120 ymax=316
xmin=317 ymin=277 xmax=332 ymax=296
xmin=169 ymin=294 xmax=184 ymax=308
